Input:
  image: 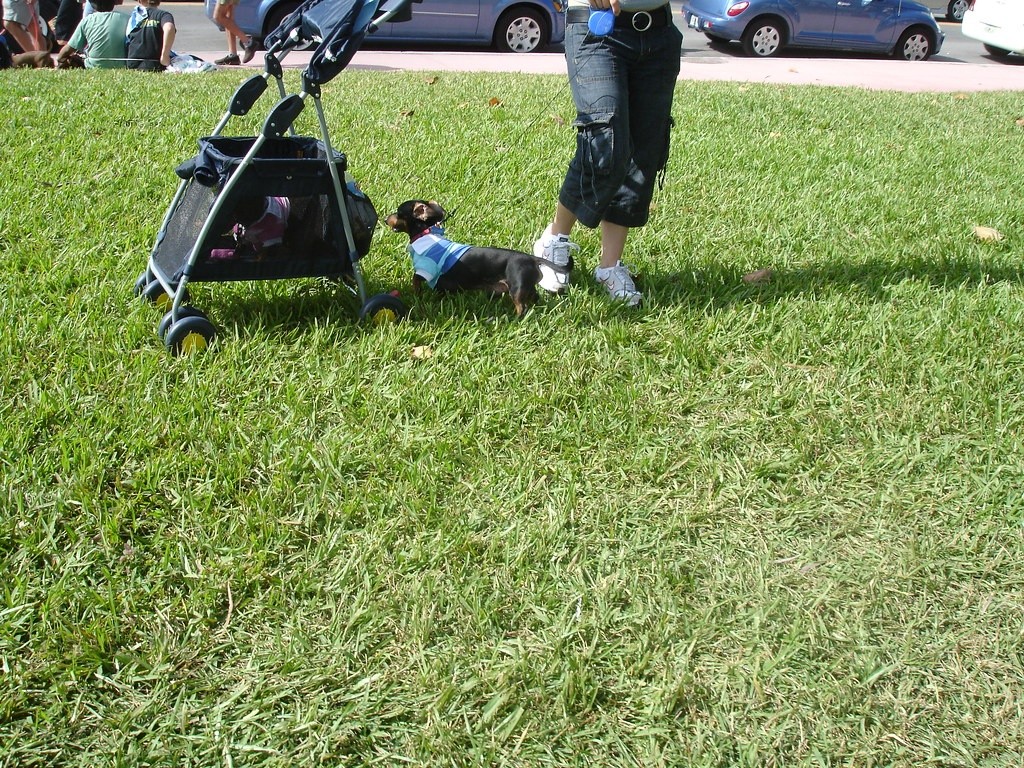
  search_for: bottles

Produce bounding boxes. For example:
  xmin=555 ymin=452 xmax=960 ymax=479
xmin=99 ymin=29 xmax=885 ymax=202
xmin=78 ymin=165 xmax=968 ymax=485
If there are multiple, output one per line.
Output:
xmin=344 ymin=169 xmax=365 ymax=213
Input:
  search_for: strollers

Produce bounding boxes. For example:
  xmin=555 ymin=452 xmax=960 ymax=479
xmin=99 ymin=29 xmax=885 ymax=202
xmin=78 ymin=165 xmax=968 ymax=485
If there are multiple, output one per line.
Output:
xmin=131 ymin=0 xmax=421 ymax=359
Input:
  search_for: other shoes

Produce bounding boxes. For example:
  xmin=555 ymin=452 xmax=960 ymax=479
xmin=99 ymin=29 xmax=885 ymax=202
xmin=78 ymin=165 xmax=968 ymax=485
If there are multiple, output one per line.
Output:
xmin=242 ymin=39 xmax=259 ymax=62
xmin=215 ymin=56 xmax=240 ymax=65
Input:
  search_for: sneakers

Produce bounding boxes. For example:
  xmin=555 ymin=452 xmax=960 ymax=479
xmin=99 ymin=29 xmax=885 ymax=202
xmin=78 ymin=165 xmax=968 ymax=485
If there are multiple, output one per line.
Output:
xmin=596 ymin=260 xmax=643 ymax=310
xmin=534 ymin=224 xmax=580 ymax=294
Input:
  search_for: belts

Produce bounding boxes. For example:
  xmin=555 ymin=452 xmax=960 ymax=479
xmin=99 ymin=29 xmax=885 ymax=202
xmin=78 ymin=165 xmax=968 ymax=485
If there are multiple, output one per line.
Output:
xmin=569 ymin=4 xmax=673 ymax=33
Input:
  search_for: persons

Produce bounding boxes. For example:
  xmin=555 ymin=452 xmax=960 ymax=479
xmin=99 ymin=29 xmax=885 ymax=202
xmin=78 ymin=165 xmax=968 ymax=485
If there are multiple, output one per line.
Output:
xmin=533 ymin=0 xmax=680 ymax=308
xmin=0 ymin=0 xmax=175 ymax=73
xmin=213 ymin=0 xmax=259 ymax=65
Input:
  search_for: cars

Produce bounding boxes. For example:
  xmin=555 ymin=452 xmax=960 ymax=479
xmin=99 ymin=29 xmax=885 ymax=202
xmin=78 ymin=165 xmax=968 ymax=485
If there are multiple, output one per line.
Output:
xmin=682 ymin=0 xmax=946 ymax=62
xmin=961 ymin=0 xmax=1024 ymax=59
xmin=203 ymin=1 xmax=570 ymax=53
xmin=917 ymin=0 xmax=973 ymax=24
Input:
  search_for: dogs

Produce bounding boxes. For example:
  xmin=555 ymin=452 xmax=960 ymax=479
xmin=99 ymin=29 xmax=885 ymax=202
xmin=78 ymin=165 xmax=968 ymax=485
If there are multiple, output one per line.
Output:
xmin=383 ymin=201 xmax=573 ymax=323
